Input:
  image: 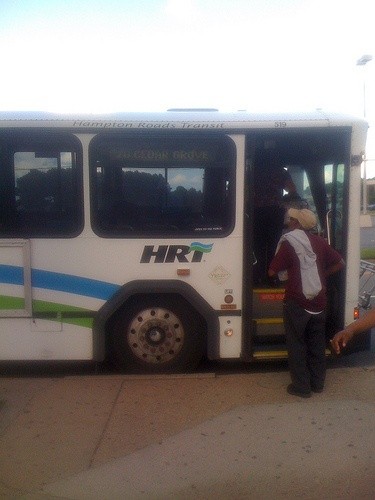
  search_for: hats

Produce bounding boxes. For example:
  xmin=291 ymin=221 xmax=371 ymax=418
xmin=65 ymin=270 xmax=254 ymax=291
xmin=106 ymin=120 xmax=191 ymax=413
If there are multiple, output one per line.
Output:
xmin=288 ymin=208 xmax=317 ymax=230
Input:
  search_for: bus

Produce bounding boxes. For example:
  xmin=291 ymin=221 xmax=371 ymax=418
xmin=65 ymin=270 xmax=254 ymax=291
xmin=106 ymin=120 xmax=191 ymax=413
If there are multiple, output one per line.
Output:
xmin=1 ymin=109 xmax=369 ymax=375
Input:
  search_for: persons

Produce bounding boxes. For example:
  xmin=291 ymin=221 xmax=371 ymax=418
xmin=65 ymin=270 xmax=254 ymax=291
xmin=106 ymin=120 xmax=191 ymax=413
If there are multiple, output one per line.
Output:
xmin=247 ymin=139 xmax=299 ymax=285
xmin=330 ymin=306 xmax=375 ymax=354
xmin=268 ymin=208 xmax=345 ymax=398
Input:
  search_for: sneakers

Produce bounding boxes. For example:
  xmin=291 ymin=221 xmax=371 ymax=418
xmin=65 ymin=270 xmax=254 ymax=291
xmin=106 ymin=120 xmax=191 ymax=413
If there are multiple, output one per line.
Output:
xmin=313 ymin=385 xmax=323 ymax=392
xmin=287 ymin=384 xmax=311 ymax=398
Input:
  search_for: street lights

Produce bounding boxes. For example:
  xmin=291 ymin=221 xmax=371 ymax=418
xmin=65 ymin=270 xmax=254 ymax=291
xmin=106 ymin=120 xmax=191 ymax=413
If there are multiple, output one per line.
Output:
xmin=357 ymin=54 xmax=372 ymax=218
